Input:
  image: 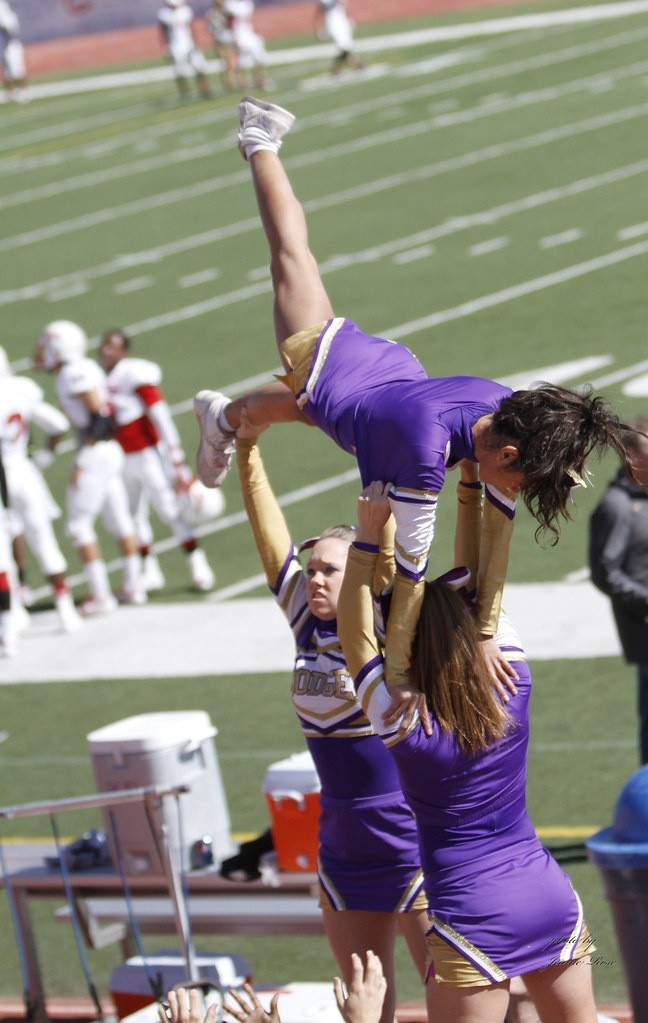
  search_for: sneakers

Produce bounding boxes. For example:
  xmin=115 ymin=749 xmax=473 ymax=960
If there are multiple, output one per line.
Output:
xmin=193 ymin=389 xmax=237 ymax=488
xmin=237 ymin=95 xmax=296 ymax=159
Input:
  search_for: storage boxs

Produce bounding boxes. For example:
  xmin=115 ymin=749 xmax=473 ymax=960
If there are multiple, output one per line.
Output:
xmin=261 ymin=749 xmax=322 ymax=872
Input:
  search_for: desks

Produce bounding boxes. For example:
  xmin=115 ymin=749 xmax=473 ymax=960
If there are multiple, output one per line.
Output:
xmin=0 ymin=852 xmax=324 ymax=1023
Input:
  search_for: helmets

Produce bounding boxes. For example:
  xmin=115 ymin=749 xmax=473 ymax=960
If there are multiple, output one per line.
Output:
xmin=178 ymin=477 xmax=225 ymax=529
xmin=40 ymin=320 xmax=87 ymax=372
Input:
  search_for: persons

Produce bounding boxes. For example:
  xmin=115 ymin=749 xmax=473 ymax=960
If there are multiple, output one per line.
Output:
xmin=157 ymin=948 xmax=389 ymax=1023
xmin=157 ymin=0 xmax=211 ymax=104
xmin=0 ymin=343 xmax=86 ymax=641
xmin=590 ymin=417 xmax=648 ymax=768
xmin=205 ymin=0 xmax=272 ymax=92
xmin=99 ymin=329 xmax=218 ymax=594
xmin=318 ymin=0 xmax=366 ymax=74
xmin=234 ymin=403 xmax=430 ymax=1023
xmin=31 ymin=318 xmax=149 ymax=613
xmin=337 ymin=459 xmax=601 ymax=1023
xmin=0 ymin=3 xmax=27 ymax=88
xmin=193 ymin=93 xmax=648 ymax=738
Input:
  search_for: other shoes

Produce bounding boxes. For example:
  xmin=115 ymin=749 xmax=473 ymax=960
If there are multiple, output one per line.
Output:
xmin=77 ymin=597 xmax=118 ymax=617
xmin=53 ymin=595 xmax=80 ymax=630
xmin=187 ymin=548 xmax=216 ymax=591
xmin=140 ymin=570 xmax=165 ymax=591
xmin=115 ymin=587 xmax=145 ymax=604
